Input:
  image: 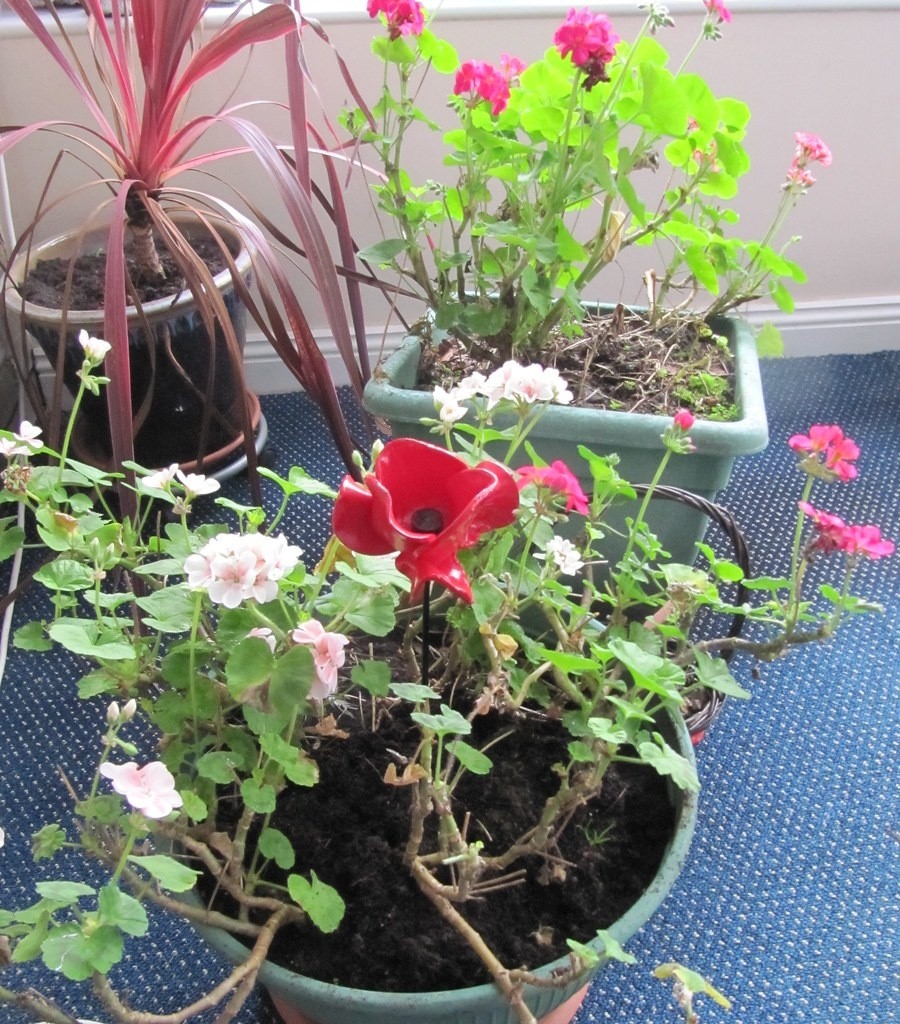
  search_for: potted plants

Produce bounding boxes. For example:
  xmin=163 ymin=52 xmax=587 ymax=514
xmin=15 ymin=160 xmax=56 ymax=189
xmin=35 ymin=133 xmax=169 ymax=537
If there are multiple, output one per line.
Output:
xmin=1 ymin=1 xmax=428 ymax=630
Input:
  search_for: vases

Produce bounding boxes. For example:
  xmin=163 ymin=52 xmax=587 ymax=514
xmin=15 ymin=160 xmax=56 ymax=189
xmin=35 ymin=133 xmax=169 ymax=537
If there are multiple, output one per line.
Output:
xmin=361 ymin=289 xmax=770 ymax=614
xmin=153 ymin=581 xmax=699 ymax=1024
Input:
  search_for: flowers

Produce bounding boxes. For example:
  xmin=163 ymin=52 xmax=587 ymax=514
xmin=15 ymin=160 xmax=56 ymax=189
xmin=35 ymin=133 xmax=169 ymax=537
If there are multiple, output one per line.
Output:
xmin=0 ymin=329 xmax=895 ymax=1024
xmin=345 ymin=2 xmax=830 ymax=423
